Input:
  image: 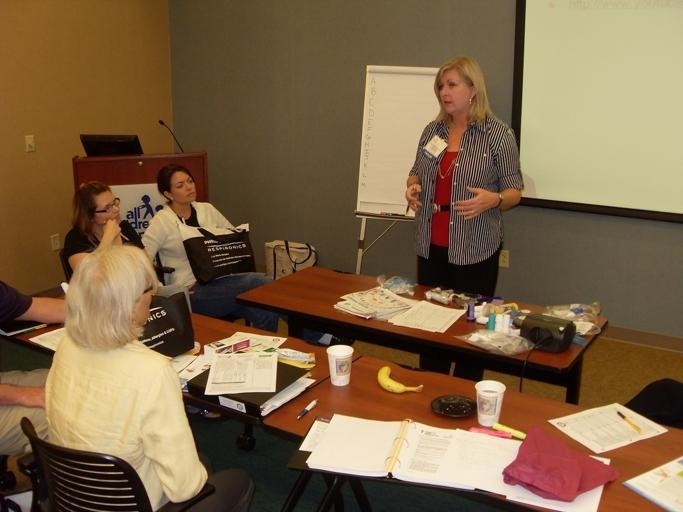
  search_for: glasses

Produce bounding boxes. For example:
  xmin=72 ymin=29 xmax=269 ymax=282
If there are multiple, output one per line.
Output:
xmin=92 ymin=197 xmax=121 ymax=214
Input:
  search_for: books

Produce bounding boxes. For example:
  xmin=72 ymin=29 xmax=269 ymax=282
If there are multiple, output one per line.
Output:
xmin=305 ymin=413 xmax=476 ymax=491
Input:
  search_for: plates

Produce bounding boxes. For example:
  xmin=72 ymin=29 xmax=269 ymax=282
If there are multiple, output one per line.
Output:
xmin=430 ymin=394 xmax=477 ymax=417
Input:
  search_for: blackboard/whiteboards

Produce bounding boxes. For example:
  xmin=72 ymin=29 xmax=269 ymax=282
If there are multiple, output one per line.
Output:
xmin=353 ymin=63 xmax=440 ymax=223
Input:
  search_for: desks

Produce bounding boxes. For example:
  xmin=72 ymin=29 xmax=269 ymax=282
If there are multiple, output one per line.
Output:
xmin=1 ymin=282 xmax=364 ymax=511
xmin=259 ymin=352 xmax=683 ymax=512
xmin=233 ymin=263 xmax=611 ymax=407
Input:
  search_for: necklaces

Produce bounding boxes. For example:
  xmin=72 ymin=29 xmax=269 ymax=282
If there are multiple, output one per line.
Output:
xmin=437 ymin=158 xmax=455 ymax=181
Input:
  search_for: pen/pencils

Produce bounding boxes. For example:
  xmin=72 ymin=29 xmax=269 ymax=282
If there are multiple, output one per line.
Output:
xmin=617 ymin=411 xmax=641 ymax=433
xmin=297 ymin=398 xmax=319 ymax=420
xmin=405 ymin=184 xmax=417 ymax=215
xmin=493 ymin=421 xmax=526 ymax=440
xmin=469 ymin=426 xmax=511 ymax=440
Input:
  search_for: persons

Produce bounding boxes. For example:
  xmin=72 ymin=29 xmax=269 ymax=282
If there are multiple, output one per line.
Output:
xmin=404 ymin=54 xmax=524 ymax=381
xmin=142 ymin=162 xmax=279 ymax=334
xmin=60 ymin=178 xmax=225 ymax=421
xmin=0 ymin=278 xmax=66 ymax=457
xmin=44 ymin=244 xmax=277 ymax=511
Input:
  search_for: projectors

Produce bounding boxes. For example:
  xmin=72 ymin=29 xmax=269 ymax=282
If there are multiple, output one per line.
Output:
xmin=520 ymin=313 xmax=576 ymax=353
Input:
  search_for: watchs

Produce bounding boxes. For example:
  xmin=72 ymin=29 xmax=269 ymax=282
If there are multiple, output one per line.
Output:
xmin=495 ymin=192 xmax=505 ymax=209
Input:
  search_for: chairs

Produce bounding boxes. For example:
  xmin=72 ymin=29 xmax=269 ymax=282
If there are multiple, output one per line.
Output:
xmin=58 ymin=246 xmax=74 ymax=284
xmin=13 ymin=415 xmax=218 ymax=511
xmin=155 ymin=266 xmax=174 ymax=286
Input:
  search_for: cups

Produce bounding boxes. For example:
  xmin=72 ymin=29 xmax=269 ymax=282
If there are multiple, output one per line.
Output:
xmin=475 ymin=380 xmax=506 ymax=427
xmin=326 ymin=345 xmax=355 ymax=387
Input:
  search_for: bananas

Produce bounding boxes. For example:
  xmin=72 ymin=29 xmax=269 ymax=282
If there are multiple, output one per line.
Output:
xmin=378 ymin=365 xmax=424 ymax=395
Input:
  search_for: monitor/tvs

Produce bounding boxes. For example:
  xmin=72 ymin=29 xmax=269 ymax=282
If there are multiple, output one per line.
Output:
xmin=80 ymin=134 xmax=144 ymax=156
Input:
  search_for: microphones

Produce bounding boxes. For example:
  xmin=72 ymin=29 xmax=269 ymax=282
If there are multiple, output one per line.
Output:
xmin=159 ymin=120 xmax=184 ymax=152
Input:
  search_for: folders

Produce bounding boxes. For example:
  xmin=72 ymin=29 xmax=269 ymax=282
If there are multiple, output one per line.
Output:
xmin=185 ymin=362 xmax=314 ymax=419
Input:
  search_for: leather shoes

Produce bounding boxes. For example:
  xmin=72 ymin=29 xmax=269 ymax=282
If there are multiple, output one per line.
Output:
xmin=305 ymin=333 xmax=355 ymax=348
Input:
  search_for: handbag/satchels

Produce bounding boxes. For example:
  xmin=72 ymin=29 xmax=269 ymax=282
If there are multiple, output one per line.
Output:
xmin=265 ymin=238 xmax=320 ymax=280
xmin=176 ymin=216 xmax=257 ymax=285
xmin=154 ymin=285 xmax=193 ymax=322
xmin=137 ymin=291 xmax=195 ymax=357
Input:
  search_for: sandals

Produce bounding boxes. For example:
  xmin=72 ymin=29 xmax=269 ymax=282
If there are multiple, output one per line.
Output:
xmin=186 ymin=407 xmax=224 ymax=422
